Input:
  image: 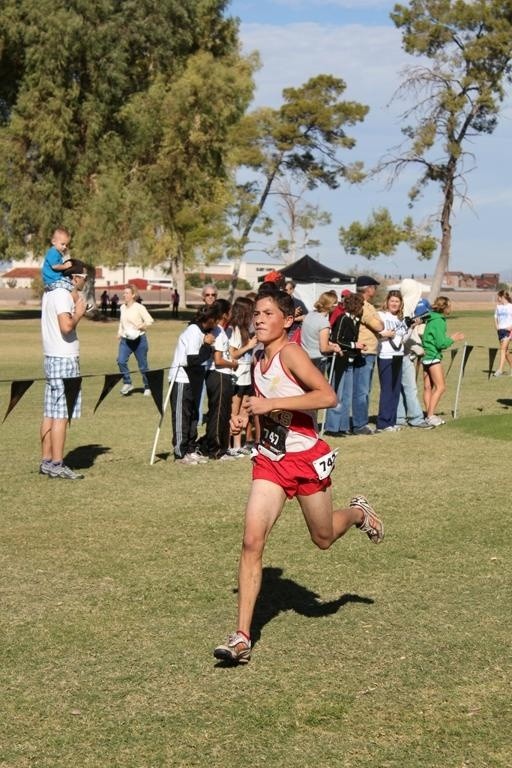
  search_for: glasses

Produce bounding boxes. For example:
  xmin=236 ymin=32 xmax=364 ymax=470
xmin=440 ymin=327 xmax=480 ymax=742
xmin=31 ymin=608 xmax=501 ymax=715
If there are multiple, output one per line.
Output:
xmin=71 ymin=273 xmax=87 ymax=280
xmin=206 ymin=294 xmax=216 ymax=296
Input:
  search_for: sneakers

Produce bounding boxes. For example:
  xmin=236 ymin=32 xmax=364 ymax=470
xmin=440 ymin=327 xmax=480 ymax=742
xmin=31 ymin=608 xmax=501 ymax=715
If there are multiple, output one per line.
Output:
xmin=494 ymin=370 xmax=502 ymax=376
xmin=120 ymin=384 xmax=133 ymax=394
xmin=350 ymin=495 xmax=384 ymax=544
xmin=49 ymin=464 xmax=84 ymax=479
xmin=174 ymin=414 xmax=445 ymax=465
xmin=40 ymin=462 xmax=49 ymax=473
xmin=215 ymin=631 xmax=251 ymax=663
xmin=144 ymin=389 xmax=151 ymax=396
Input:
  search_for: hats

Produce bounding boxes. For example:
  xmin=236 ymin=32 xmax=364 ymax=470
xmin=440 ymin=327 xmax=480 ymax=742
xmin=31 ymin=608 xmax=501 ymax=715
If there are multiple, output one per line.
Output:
xmin=357 ymin=276 xmax=379 ymax=286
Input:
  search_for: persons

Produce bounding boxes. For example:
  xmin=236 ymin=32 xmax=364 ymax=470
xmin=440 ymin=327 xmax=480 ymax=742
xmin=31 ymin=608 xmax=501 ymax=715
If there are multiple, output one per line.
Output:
xmin=211 ymin=289 xmax=384 ymax=664
xmin=110 ymin=294 xmax=119 ymax=318
xmin=493 ymin=290 xmax=512 ymax=377
xmin=101 ymin=290 xmax=110 ymax=315
xmin=39 ymin=259 xmax=87 ymax=479
xmin=41 ymin=227 xmax=95 ymax=312
xmin=115 ymin=286 xmax=154 ymax=396
xmin=169 ymin=275 xmax=466 ymax=464
